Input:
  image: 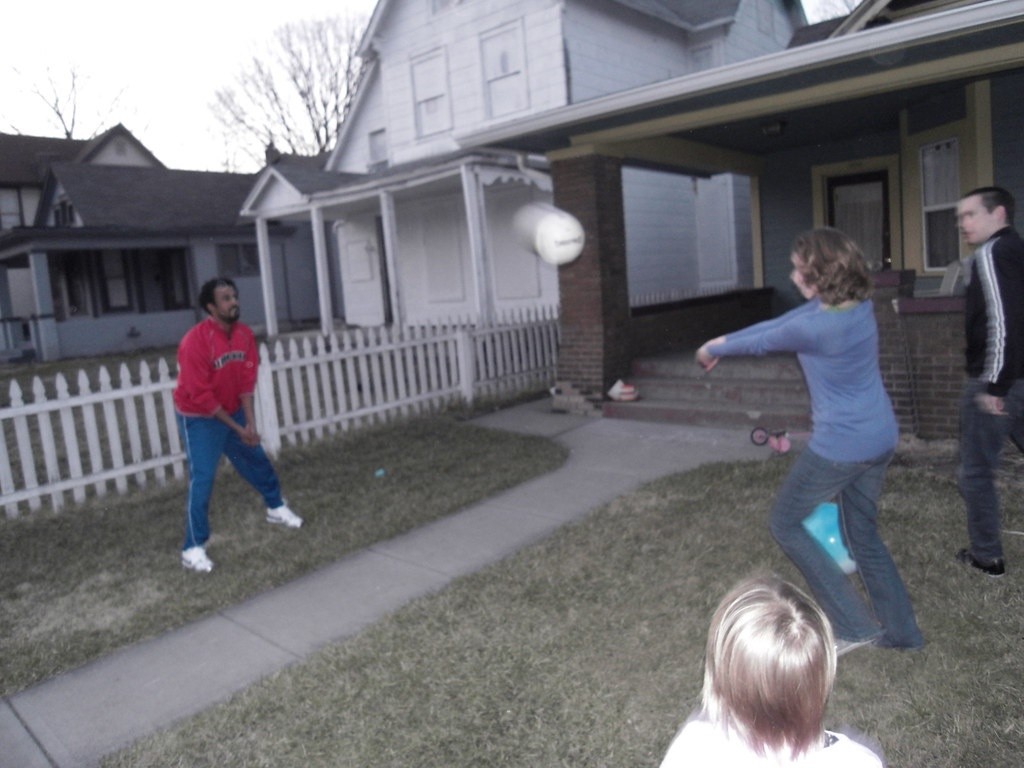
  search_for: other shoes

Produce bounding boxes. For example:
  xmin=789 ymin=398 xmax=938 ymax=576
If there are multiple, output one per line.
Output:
xmin=954 ymin=548 xmax=1006 ymax=578
xmin=871 ymin=636 xmax=924 ymax=650
xmin=832 ymin=631 xmax=878 ymax=658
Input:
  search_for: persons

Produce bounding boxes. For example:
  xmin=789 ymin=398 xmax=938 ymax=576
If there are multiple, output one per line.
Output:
xmin=955 ymin=187 xmax=1024 ymax=577
xmin=176 ymin=275 xmax=303 ymax=572
xmin=695 ymin=227 xmax=928 ymax=656
xmin=656 ymin=577 xmax=883 ymax=768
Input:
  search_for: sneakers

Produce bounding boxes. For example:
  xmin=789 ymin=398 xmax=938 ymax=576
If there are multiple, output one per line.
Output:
xmin=181 ymin=546 xmax=214 ymax=573
xmin=266 ymin=497 xmax=305 ymax=528
